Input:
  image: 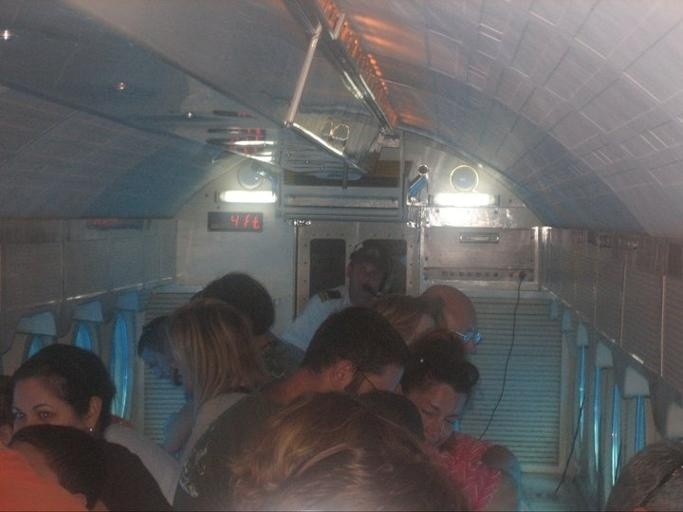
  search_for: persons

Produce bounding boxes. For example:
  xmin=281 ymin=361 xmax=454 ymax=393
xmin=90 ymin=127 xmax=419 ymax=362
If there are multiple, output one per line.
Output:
xmin=603 ymin=436 xmax=683 ymax=512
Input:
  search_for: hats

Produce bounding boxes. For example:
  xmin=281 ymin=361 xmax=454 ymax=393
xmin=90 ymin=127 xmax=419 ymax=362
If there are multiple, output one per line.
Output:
xmin=349 ymin=248 xmax=383 ymax=262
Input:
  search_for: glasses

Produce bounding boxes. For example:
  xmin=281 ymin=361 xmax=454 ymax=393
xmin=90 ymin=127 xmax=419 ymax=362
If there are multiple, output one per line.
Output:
xmin=452 ymin=329 xmax=481 ymax=343
